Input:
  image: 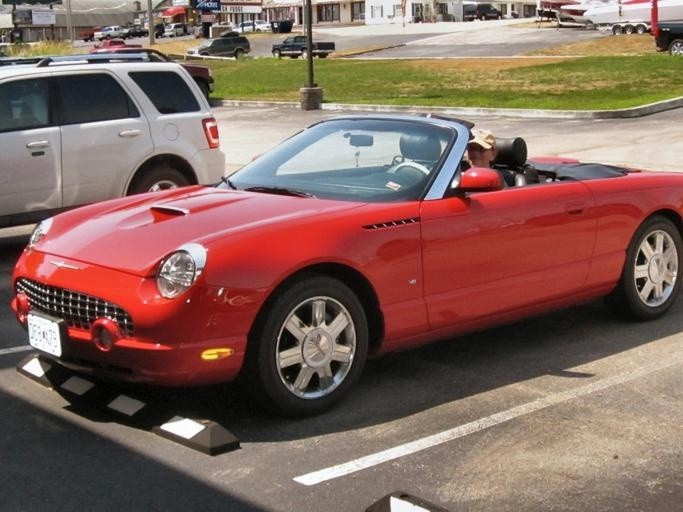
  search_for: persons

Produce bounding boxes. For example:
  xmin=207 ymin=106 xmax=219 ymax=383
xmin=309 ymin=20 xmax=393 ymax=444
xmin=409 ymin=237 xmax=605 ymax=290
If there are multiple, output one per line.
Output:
xmin=10 ymin=22 xmax=22 ymax=43
xmin=465 ymin=130 xmax=509 ymax=191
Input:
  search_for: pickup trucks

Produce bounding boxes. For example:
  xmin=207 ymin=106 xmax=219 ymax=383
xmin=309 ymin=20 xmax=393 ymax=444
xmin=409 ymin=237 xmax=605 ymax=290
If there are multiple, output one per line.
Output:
xmin=272 ymin=36 xmax=335 ymax=59
xmin=87 ymin=40 xmax=142 ymax=53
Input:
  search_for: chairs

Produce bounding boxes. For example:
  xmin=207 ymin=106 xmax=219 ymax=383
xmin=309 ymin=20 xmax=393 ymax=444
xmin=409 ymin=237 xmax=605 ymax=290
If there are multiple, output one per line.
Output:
xmin=487 ymin=136 xmax=540 ymax=186
xmin=389 ymin=131 xmax=441 ymax=183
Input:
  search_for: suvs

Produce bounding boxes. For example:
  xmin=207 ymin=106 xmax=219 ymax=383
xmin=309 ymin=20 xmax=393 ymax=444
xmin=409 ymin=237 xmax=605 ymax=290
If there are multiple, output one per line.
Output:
xmin=0 ymin=48 xmax=225 ymax=230
xmin=90 ymin=49 xmax=215 ymax=103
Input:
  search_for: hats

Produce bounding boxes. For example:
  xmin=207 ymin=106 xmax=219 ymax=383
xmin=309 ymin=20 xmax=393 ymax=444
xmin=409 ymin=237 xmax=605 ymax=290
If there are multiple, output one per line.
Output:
xmin=467 ymin=128 xmax=497 ymax=151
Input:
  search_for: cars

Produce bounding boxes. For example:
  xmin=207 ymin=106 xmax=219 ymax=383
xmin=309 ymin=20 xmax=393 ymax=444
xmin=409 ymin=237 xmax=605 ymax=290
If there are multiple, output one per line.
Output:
xmin=655 ymin=19 xmax=683 ymax=55
xmin=463 ymin=2 xmax=502 ymax=21
xmin=186 ymin=36 xmax=250 ymax=59
xmin=80 ymin=20 xmax=268 ymax=41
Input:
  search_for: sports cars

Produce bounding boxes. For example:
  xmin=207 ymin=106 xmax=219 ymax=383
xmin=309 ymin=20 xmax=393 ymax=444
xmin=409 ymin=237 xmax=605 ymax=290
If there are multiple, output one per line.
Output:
xmin=8 ymin=113 xmax=683 ymax=417
